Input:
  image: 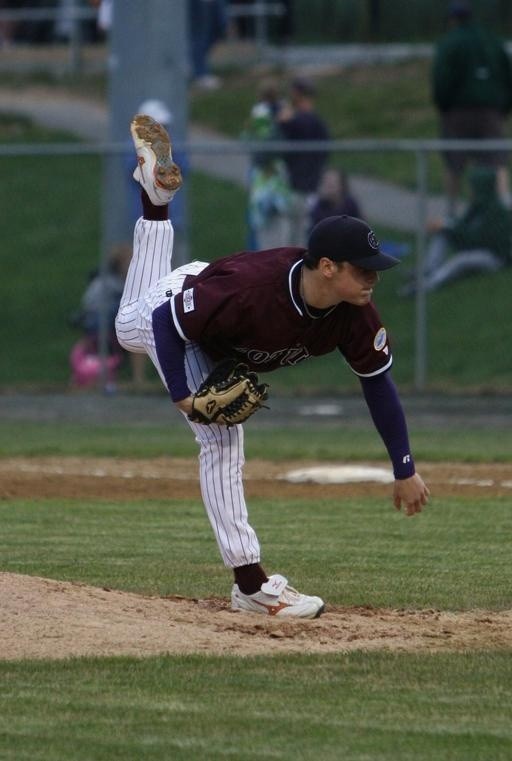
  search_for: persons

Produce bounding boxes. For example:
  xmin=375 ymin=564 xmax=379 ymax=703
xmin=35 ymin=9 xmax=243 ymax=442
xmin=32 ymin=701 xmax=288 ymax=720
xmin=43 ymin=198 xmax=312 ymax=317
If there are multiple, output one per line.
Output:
xmin=431 ymin=1 xmax=512 ymax=223
xmin=78 ymin=242 xmax=153 ymax=393
xmin=394 ymin=166 xmax=512 ymax=301
xmin=112 ymin=113 xmax=432 ymax=620
xmin=245 ymin=77 xmax=359 ymax=251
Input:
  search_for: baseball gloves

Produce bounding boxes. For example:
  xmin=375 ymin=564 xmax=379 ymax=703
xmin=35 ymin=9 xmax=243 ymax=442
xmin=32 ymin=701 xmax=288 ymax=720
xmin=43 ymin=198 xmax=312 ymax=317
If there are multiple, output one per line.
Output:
xmin=188 ymin=359 xmax=269 ymax=431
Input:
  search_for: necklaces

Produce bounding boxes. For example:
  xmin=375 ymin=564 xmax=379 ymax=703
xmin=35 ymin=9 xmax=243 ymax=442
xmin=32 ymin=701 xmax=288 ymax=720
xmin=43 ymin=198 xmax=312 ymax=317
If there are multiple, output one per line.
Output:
xmin=300 ymin=264 xmax=338 ymax=322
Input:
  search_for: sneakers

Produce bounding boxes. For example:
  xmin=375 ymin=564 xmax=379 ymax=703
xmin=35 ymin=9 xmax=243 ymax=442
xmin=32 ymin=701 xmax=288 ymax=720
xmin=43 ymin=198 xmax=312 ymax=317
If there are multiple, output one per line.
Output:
xmin=130 ymin=113 xmax=182 ymax=208
xmin=231 ymin=572 xmax=326 ymax=620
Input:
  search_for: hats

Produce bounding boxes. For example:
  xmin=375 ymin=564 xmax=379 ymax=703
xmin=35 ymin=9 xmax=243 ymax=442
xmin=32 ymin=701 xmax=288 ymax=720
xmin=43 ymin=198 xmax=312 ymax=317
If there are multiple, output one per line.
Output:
xmin=306 ymin=212 xmax=401 ymax=272
